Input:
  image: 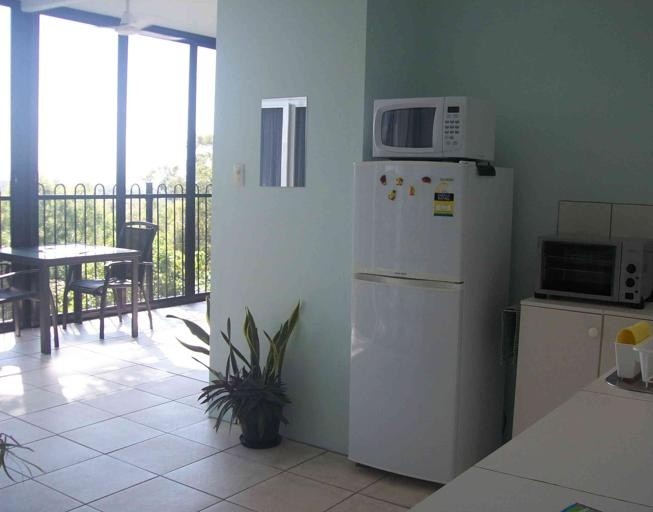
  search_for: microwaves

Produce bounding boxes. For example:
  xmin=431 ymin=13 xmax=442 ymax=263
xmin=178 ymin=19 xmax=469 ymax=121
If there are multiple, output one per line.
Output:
xmin=371 ymin=96 xmax=496 ymax=163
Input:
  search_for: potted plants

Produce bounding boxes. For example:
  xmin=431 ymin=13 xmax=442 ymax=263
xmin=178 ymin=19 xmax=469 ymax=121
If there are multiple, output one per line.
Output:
xmin=192 ymin=296 xmax=304 ymax=450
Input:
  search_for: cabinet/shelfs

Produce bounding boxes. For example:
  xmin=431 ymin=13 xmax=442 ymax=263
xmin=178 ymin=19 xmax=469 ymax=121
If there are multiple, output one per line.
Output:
xmin=510 ymin=300 xmax=651 ymax=439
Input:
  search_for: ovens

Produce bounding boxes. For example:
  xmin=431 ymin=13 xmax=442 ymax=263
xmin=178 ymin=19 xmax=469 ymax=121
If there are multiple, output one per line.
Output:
xmin=534 ymin=234 xmax=652 ymax=310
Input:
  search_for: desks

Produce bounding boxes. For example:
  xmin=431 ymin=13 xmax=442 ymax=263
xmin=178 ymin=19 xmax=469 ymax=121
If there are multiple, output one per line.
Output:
xmin=0 ymin=244 xmax=140 ymax=356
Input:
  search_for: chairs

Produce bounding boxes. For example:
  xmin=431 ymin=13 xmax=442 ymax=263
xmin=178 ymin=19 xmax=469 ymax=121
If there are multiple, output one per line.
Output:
xmin=63 ymin=218 xmax=158 ymax=339
xmin=0 ymin=261 xmax=60 ymax=348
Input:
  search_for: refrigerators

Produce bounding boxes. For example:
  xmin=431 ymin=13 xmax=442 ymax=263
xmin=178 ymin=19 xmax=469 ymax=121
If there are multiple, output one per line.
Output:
xmin=345 ymin=161 xmax=516 ymax=487
